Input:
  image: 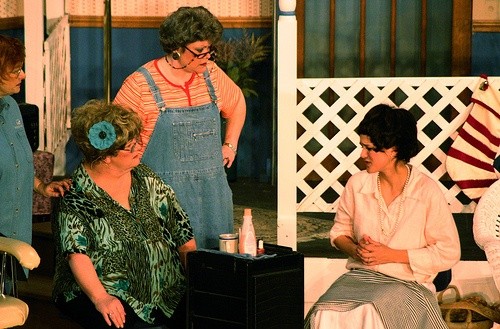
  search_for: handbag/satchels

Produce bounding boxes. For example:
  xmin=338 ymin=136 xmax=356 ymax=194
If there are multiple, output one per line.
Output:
xmin=437 ymin=285 xmax=500 ymax=329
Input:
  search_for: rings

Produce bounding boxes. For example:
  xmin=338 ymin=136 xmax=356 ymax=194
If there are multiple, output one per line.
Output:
xmin=108 ymin=312 xmax=113 ymax=316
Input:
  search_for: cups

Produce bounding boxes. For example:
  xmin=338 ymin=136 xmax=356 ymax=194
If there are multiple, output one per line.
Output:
xmin=218 ymin=238 xmax=238 ymax=254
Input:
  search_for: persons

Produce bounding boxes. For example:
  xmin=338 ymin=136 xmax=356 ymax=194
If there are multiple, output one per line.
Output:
xmin=0 ymin=34 xmax=72 ymax=296
xmin=109 ymin=6 xmax=246 ymax=249
xmin=54 ymin=99 xmax=197 ymax=329
xmin=304 ymin=107 xmax=461 ymax=329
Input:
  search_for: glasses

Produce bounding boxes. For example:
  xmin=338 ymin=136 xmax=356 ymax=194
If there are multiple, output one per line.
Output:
xmin=6 ymin=68 xmax=22 ymax=76
xmin=117 ymin=136 xmax=139 ymax=153
xmin=183 ymin=45 xmax=216 ymax=59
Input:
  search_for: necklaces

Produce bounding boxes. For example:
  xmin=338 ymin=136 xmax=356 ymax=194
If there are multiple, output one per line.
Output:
xmin=377 ymin=164 xmax=410 ymax=236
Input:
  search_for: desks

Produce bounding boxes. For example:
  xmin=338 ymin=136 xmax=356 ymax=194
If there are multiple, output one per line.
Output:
xmin=187 ymin=241 xmax=304 ymax=329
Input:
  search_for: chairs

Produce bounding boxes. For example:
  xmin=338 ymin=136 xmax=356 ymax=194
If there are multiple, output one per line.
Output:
xmin=0 ymin=236 xmax=41 ymax=329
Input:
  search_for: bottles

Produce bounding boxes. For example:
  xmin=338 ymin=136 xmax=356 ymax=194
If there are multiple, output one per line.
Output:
xmin=239 ymin=208 xmax=256 ymax=256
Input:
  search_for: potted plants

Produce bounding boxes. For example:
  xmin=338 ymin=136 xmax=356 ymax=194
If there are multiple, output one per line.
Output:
xmin=210 ymin=26 xmax=272 ymax=181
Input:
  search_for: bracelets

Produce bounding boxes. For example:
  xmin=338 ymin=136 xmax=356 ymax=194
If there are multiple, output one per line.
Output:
xmin=223 ymin=142 xmax=237 ymax=155
